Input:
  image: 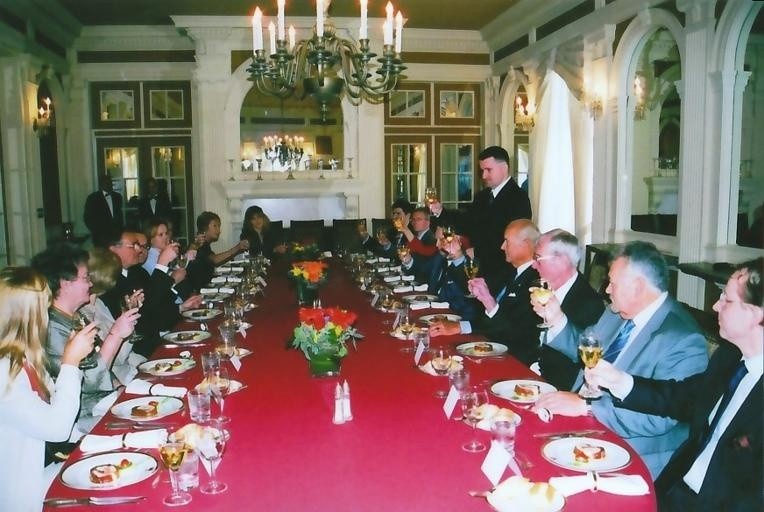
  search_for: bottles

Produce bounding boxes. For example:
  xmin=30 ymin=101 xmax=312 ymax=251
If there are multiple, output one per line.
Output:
xmin=331 ymin=380 xmax=344 ymax=426
xmin=312 ymin=298 xmax=323 ymax=309
xmin=338 ymin=380 xmax=353 ymax=420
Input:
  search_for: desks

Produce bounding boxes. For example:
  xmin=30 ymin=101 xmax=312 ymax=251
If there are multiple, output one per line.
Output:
xmin=582 ymin=241 xmax=679 ymax=299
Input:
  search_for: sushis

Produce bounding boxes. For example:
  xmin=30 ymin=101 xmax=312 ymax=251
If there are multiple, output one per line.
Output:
xmin=131 ymin=404 xmax=155 ymax=418
xmin=513 ymin=384 xmax=542 ymax=398
xmin=473 ymin=342 xmax=493 ymax=353
xmin=154 ymin=360 xmax=172 ymax=373
xmin=89 ymin=464 xmax=118 ymax=483
xmin=204 ymin=293 xmax=217 ymax=300
xmin=434 ymin=315 xmax=447 ymax=322
xmin=415 ymin=296 xmax=429 ymax=301
xmin=573 ymin=442 xmax=605 ymax=463
xmin=193 ymin=312 xmax=207 ymax=317
xmin=177 ymin=334 xmax=194 ymax=340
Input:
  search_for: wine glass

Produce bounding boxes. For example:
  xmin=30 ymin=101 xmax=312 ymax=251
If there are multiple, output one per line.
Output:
xmin=356 ymin=185 xmax=459 ymax=262
xmin=576 ymin=329 xmax=607 ymax=401
xmin=448 ymin=366 xmax=470 ymax=420
xmin=157 ymin=231 xmax=265 ymax=508
xmin=427 ymin=345 xmax=450 ymax=401
xmin=352 ymin=264 xmax=378 ymax=303
xmin=462 ymin=257 xmax=481 ymax=299
xmin=117 ymin=297 xmax=149 ymax=343
xmin=531 ymin=276 xmax=552 ymax=329
xmin=71 ymin=312 xmax=98 ymax=372
xmin=459 ymin=384 xmax=488 ymax=454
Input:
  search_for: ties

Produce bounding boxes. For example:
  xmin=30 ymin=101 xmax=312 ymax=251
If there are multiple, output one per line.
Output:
xmin=601 ymin=319 xmax=635 ymax=363
xmin=695 ymin=360 xmax=748 ymax=454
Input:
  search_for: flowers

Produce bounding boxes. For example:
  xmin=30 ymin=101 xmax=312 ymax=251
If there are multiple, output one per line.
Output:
xmin=286 ymin=303 xmax=364 ymax=361
xmin=284 ymin=232 xmax=333 ymax=289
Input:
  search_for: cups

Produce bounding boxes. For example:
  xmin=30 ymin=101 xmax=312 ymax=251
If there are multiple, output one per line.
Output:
xmin=489 ymin=414 xmax=516 ymax=454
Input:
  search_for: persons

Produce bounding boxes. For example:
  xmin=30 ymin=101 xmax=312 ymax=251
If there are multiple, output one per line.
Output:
xmin=83 ymin=174 xmax=123 ymax=246
xmin=429 ymin=145 xmax=532 ymax=244
xmin=137 ymin=177 xmax=172 ymax=217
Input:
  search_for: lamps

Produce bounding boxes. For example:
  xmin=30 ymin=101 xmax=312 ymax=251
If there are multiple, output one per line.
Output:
xmin=631 ymin=76 xmax=648 ymax=122
xmin=244 ymin=0 xmax=410 ymax=123
xmin=515 ymin=95 xmax=537 ymax=133
xmin=33 ymin=97 xmax=52 ymax=138
xmin=264 ymin=132 xmax=306 ymax=182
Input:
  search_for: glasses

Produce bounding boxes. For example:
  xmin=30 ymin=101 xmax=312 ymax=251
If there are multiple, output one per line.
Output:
xmin=140 ymin=243 xmax=150 ymax=250
xmin=115 ymin=243 xmax=140 ymax=248
xmin=77 ymin=275 xmax=91 ymax=284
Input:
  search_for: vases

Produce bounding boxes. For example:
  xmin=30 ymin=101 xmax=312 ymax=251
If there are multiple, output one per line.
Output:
xmin=294 ymin=275 xmax=320 ymax=307
xmin=308 ymin=344 xmax=343 ymax=379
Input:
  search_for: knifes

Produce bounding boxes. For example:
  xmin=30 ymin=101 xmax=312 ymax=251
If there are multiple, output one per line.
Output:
xmin=43 ymin=494 xmax=148 ymax=507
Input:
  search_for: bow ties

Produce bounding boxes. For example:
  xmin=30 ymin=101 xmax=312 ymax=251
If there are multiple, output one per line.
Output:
xmin=149 ymin=196 xmax=159 ymax=201
xmin=106 ymin=193 xmax=112 ymax=196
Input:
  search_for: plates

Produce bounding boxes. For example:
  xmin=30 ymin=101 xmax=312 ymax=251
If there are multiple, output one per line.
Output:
xmin=464 ymin=409 xmax=524 ymax=431
xmin=486 ymin=482 xmax=565 ymax=512
xmin=378 ymin=270 xmax=510 ymax=357
xmin=490 ymin=381 xmax=561 ymax=407
xmin=540 ymin=437 xmax=633 ymax=475
xmin=59 ymin=360 xmax=157 ymax=494
